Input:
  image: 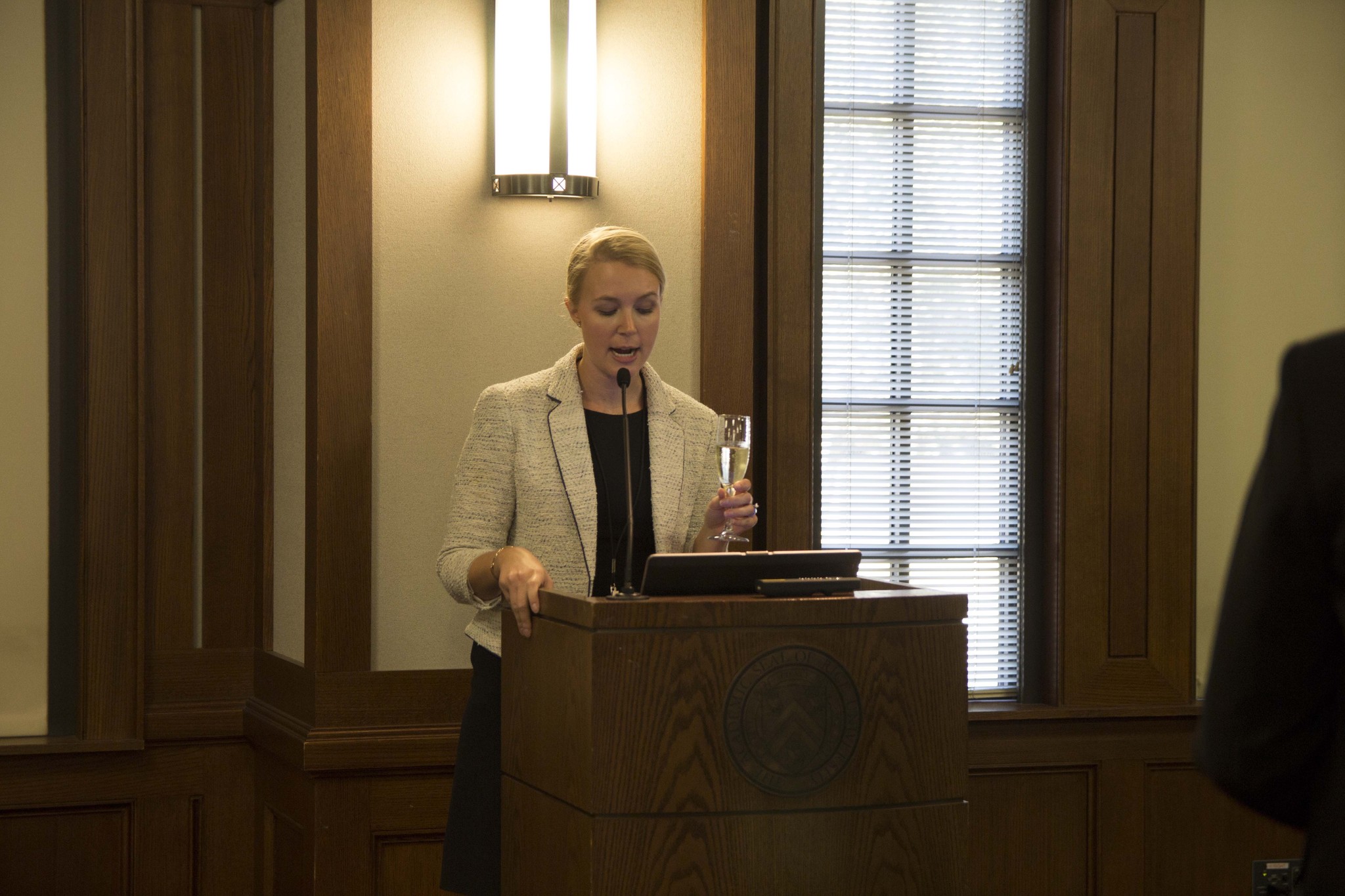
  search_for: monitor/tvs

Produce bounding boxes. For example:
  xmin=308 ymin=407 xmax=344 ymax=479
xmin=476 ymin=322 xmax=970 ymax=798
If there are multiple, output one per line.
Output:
xmin=636 ymin=549 xmax=862 ymax=594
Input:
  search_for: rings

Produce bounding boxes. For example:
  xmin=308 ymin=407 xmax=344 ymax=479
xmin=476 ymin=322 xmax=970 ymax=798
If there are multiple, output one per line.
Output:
xmin=750 ymin=502 xmax=759 ymax=518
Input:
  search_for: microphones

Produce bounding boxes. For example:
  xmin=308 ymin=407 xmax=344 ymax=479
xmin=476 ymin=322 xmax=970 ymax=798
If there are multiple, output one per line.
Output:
xmin=607 ymin=367 xmax=648 ymax=600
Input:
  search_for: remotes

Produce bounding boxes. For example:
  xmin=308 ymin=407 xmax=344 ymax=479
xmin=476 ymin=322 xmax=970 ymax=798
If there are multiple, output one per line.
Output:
xmin=757 ymin=576 xmax=862 ymax=592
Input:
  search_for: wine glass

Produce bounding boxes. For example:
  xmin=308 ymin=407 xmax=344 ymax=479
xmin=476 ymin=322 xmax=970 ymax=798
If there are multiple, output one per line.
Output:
xmin=708 ymin=413 xmax=752 ymax=543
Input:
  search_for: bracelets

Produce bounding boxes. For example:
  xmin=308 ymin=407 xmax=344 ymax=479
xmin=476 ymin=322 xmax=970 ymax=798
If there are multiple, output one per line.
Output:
xmin=489 ymin=545 xmax=515 ymax=581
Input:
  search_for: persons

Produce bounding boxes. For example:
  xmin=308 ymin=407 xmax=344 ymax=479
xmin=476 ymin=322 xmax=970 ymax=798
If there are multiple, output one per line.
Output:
xmin=434 ymin=224 xmax=760 ymax=895
xmin=1190 ymin=322 xmax=1345 ymax=896
